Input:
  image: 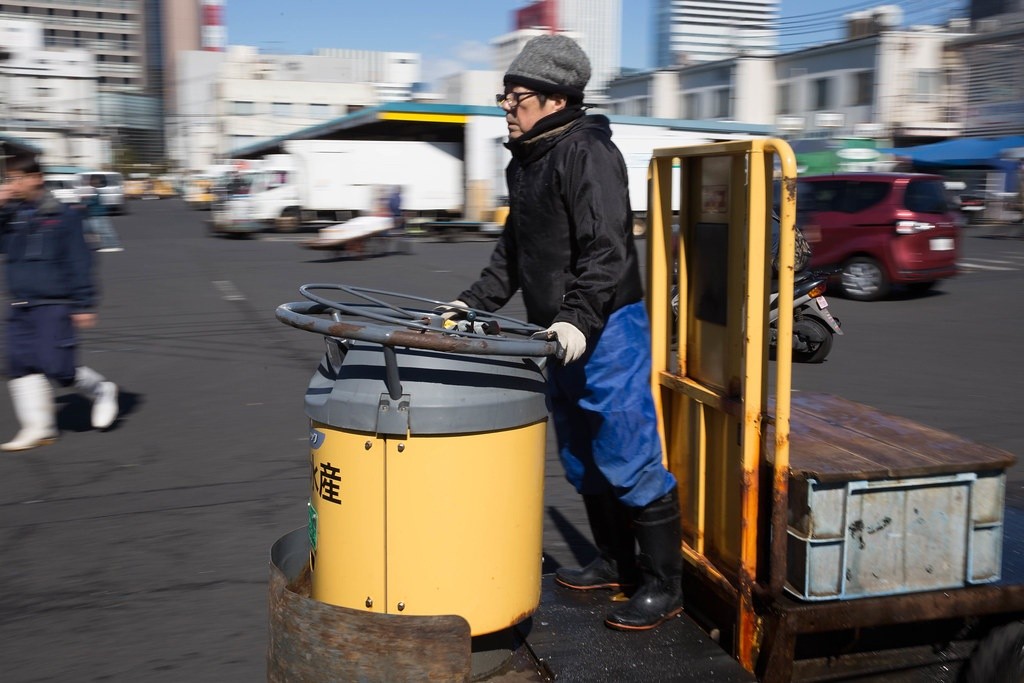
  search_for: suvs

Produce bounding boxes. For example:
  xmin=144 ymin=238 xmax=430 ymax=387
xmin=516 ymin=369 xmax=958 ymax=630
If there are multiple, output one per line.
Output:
xmin=772 ymin=172 xmax=963 ymax=302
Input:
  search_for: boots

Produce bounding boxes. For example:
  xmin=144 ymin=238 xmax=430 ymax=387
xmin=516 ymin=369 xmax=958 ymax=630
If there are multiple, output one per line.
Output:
xmin=555 ymin=495 xmax=634 ymax=589
xmin=0 ymin=374 xmax=59 ymax=451
xmin=603 ymin=483 xmax=684 ymax=630
xmin=72 ymin=366 xmax=119 ymax=429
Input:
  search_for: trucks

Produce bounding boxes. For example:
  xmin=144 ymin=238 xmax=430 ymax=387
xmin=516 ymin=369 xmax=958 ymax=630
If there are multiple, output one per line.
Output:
xmin=211 ymin=134 xmax=466 ymax=235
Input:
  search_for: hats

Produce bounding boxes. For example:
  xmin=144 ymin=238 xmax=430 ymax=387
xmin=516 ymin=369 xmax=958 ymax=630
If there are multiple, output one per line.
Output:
xmin=504 ymin=35 xmax=591 ymax=100
xmin=0 ymin=139 xmax=44 ymax=171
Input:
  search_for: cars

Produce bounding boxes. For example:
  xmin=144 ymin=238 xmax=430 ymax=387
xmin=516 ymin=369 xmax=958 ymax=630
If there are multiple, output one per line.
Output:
xmin=42 ymin=159 xmax=229 ymax=213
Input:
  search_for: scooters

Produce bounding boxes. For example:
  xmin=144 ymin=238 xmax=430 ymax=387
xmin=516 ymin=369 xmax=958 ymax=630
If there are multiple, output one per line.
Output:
xmin=666 ymin=225 xmax=846 ymax=364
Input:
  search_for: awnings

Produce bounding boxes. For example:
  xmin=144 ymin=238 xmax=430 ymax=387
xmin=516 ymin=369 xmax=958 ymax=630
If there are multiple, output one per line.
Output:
xmin=879 ymin=133 xmax=1024 ymax=165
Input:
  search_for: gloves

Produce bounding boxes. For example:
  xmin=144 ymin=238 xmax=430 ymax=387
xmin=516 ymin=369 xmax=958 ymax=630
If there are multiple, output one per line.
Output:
xmin=430 ymin=300 xmax=469 ymax=320
xmin=528 ymin=322 xmax=587 ymax=367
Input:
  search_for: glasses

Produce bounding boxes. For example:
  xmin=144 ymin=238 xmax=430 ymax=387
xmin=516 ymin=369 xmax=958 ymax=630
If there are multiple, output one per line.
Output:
xmin=496 ymin=91 xmax=551 ymax=107
xmin=0 ymin=174 xmax=37 ymax=184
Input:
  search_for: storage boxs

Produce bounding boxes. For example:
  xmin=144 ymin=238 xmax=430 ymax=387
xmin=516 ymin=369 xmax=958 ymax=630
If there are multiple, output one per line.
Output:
xmin=759 ymin=464 xmax=1008 ymax=602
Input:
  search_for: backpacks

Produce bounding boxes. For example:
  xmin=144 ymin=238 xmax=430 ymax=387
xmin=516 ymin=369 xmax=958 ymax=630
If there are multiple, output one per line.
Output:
xmin=770 ymin=214 xmax=812 ymax=273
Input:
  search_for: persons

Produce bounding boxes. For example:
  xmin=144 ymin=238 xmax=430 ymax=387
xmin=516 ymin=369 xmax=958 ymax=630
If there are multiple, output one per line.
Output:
xmin=0 ymin=157 xmax=121 ymax=451
xmin=430 ymin=34 xmax=683 ymax=629
